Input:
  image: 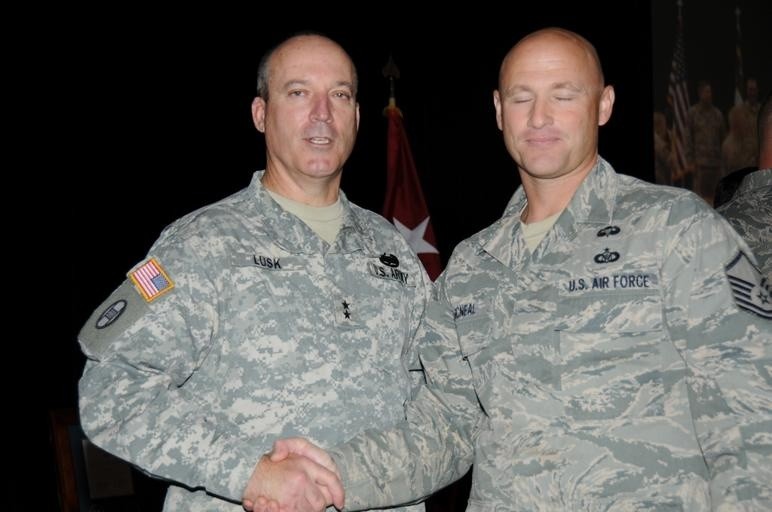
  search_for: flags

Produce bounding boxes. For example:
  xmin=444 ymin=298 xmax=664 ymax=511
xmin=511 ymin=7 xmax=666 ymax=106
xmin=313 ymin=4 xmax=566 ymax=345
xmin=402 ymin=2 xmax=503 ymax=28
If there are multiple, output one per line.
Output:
xmin=662 ymin=26 xmax=696 ymax=188
xmin=380 ymin=97 xmax=442 ymax=288
xmin=728 ymin=8 xmax=747 ymax=112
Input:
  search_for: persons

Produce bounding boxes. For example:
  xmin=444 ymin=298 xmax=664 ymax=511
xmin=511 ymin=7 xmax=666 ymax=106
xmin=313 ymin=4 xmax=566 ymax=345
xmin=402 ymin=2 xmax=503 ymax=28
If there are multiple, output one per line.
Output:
xmin=654 ymin=80 xmax=763 ymax=209
xmin=712 ymin=91 xmax=771 ymax=292
xmin=69 ymin=30 xmax=439 ymax=512
xmin=237 ymin=22 xmax=770 ymax=512
